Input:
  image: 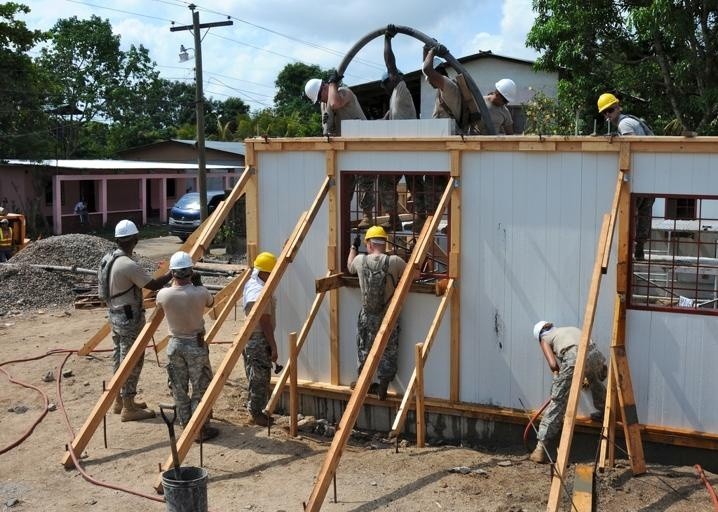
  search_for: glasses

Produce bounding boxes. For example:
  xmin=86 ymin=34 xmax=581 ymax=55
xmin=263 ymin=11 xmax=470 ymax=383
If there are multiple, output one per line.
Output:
xmin=603 ymin=105 xmax=615 ymax=114
xmin=501 ymin=96 xmax=508 ymax=103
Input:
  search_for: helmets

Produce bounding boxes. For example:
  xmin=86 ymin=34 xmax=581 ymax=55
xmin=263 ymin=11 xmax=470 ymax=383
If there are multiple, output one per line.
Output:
xmin=495 ymin=78 xmax=516 ymax=102
xmin=381 ymin=67 xmax=403 ymax=82
xmin=533 ymin=320 xmax=553 ymax=342
xmin=597 ymin=92 xmax=620 ymax=114
xmin=254 ymin=252 xmax=277 ymax=273
xmin=304 ymin=78 xmax=323 ymax=104
xmin=114 ymin=219 xmax=139 ymax=238
xmin=169 ymin=251 xmax=194 ymax=269
xmin=421 ymin=57 xmax=443 ymax=77
xmin=363 ymin=226 xmax=387 ymax=241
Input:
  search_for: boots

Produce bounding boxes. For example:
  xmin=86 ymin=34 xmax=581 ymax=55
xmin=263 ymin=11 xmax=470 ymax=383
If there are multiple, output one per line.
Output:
xmin=120 ymin=397 xmax=156 ymax=421
xmin=378 ymin=380 xmax=389 ymax=400
xmin=632 ymin=243 xmax=643 ymax=259
xmin=528 ymin=442 xmax=548 ymax=463
xmin=591 ymin=411 xmax=604 ymax=421
xmin=381 ymin=213 xmax=402 ymax=231
xmin=358 ymin=208 xmax=374 ymax=228
xmin=350 ymin=381 xmax=357 ymax=389
xmin=113 ymin=392 xmax=147 ymax=415
xmin=404 ymin=213 xmax=427 ymax=231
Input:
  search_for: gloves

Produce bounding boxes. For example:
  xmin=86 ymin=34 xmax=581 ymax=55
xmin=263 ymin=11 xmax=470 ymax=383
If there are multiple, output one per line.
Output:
xmin=353 ymin=235 xmax=361 ymax=248
xmin=328 ymin=69 xmax=345 ymax=85
xmin=190 ymin=272 xmax=203 ymax=287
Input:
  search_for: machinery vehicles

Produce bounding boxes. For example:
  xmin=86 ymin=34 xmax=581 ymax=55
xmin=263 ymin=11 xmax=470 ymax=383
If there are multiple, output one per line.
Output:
xmin=0 ymin=206 xmax=42 ymax=258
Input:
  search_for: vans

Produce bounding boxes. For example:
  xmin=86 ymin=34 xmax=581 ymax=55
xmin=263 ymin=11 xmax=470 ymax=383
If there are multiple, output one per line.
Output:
xmin=166 ymin=190 xmax=231 ymax=244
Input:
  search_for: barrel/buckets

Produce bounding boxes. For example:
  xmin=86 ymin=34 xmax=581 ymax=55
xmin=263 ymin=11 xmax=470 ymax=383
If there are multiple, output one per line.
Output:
xmin=162 ymin=465 xmax=209 ymax=512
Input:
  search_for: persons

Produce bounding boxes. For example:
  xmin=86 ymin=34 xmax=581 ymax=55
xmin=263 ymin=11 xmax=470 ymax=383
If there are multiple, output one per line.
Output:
xmin=0 ymin=217 xmax=15 ymax=263
xmin=467 ymin=76 xmax=516 ymax=136
xmin=240 ymin=249 xmax=280 ymax=427
xmin=153 ymin=249 xmax=224 ymax=444
xmin=524 ymin=316 xmax=614 ymax=463
xmin=96 ymin=219 xmax=175 ymax=424
xmin=302 ymin=70 xmax=370 ymax=137
xmin=374 ymin=21 xmax=419 ymax=121
xmin=345 ymin=225 xmax=421 ymax=400
xmin=71 ymin=198 xmax=92 ymax=226
xmin=594 ymin=90 xmax=658 ymax=264
xmin=419 ymin=37 xmax=473 ymax=136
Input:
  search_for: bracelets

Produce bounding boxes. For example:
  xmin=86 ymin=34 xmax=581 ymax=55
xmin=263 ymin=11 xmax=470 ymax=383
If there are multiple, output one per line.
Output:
xmin=326 ymin=79 xmax=336 ymax=85
xmin=348 ymin=246 xmax=358 ymax=252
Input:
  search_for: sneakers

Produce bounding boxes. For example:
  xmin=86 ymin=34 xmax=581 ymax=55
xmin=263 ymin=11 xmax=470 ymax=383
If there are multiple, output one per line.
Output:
xmin=262 ymin=414 xmax=274 ymax=421
xmin=249 ymin=415 xmax=273 ymax=426
xmin=195 ymin=428 xmax=219 ymax=443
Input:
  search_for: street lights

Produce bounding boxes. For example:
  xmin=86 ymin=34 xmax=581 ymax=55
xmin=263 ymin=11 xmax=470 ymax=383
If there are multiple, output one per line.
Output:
xmin=176 ymin=40 xmax=208 ymax=228
